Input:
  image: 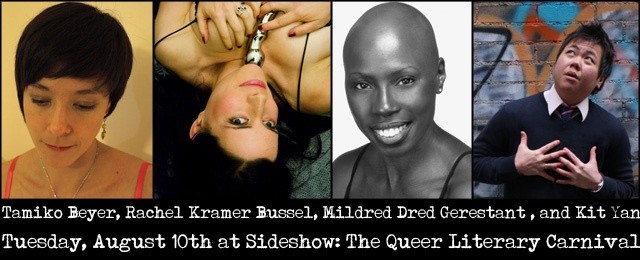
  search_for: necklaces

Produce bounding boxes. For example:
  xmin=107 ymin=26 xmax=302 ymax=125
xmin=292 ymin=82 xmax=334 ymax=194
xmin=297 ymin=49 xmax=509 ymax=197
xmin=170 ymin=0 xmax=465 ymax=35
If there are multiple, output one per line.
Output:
xmin=37 ymin=138 xmax=100 ymax=200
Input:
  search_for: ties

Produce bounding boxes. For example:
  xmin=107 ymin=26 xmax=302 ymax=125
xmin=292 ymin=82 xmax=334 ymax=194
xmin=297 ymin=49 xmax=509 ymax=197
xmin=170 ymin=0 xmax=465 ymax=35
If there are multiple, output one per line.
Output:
xmin=558 ymin=105 xmax=578 ymax=118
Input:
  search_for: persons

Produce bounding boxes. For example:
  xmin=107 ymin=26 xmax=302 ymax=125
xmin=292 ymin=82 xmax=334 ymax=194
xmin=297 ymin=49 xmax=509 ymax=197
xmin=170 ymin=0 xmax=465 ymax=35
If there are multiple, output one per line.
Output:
xmin=471 ymin=21 xmax=634 ymax=199
xmin=2 ymin=2 xmax=154 ymax=199
xmin=153 ymin=2 xmax=332 ymax=199
xmin=332 ymin=1 xmax=472 ymax=200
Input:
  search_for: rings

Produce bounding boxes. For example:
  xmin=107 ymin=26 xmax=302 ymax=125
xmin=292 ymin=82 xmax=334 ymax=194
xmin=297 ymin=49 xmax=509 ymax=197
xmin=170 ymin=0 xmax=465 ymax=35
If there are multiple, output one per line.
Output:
xmin=235 ymin=6 xmax=247 ymax=16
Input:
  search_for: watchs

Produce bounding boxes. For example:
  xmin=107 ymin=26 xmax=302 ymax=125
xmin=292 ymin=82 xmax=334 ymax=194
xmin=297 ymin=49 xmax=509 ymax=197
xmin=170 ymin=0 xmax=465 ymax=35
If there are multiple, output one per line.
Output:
xmin=591 ymin=178 xmax=604 ymax=194
